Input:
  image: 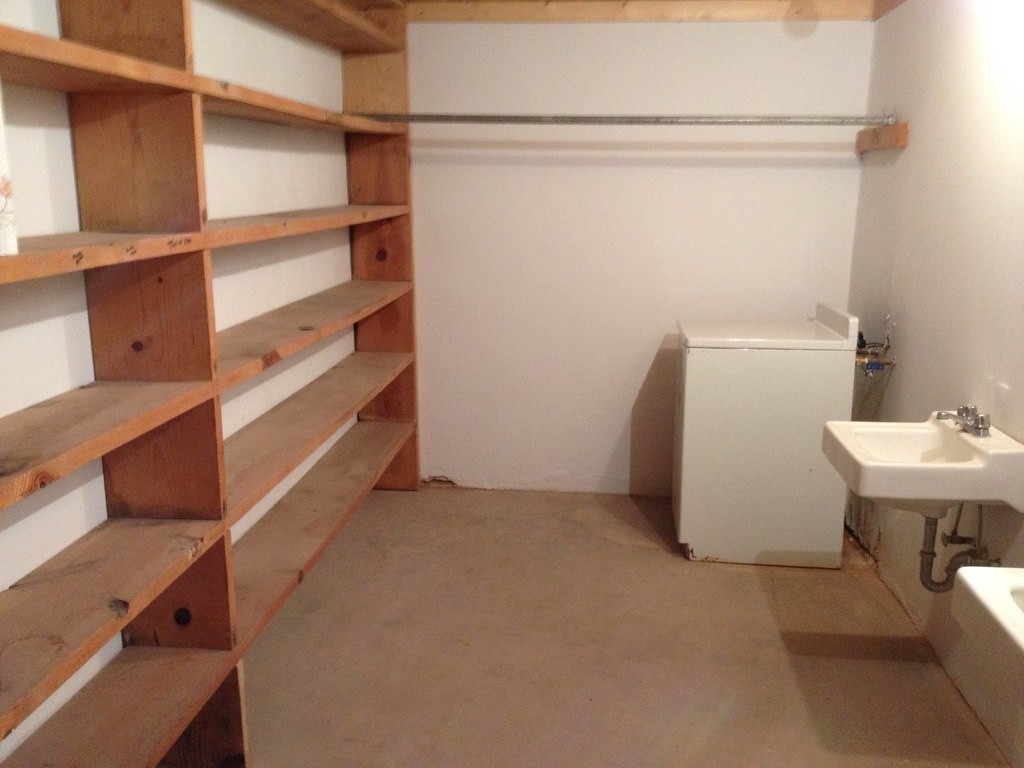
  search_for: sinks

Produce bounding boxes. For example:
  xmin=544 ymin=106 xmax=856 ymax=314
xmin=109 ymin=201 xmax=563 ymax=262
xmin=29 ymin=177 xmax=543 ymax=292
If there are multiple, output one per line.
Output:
xmin=822 ymin=410 xmax=1024 ymax=516
xmin=951 ymin=566 xmax=1024 ymax=670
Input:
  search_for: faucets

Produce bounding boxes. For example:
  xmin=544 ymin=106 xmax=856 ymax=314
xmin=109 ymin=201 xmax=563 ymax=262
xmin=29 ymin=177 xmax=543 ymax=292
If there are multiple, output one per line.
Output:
xmin=936 ymin=405 xmax=991 ymax=438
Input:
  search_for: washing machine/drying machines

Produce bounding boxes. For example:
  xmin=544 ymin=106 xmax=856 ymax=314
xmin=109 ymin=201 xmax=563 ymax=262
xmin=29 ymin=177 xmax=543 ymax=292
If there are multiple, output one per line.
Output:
xmin=671 ymin=304 xmax=859 ymax=570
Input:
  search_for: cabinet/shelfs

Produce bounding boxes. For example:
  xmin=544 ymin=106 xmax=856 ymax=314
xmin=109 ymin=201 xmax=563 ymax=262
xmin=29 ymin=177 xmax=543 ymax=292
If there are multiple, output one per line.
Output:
xmin=0 ymin=0 xmax=421 ymax=768
